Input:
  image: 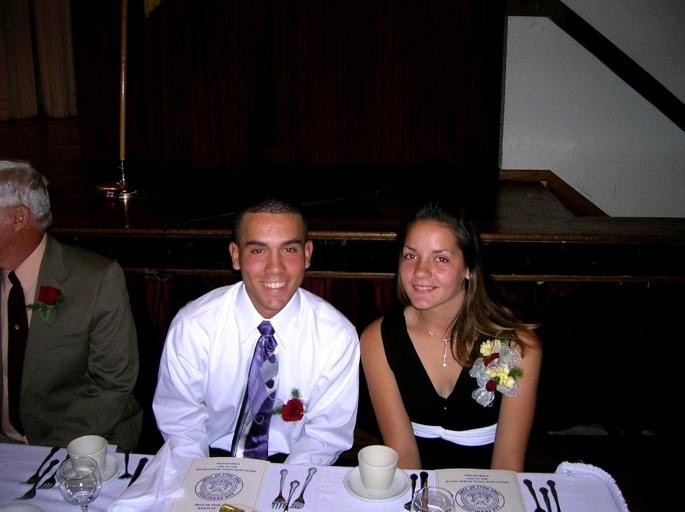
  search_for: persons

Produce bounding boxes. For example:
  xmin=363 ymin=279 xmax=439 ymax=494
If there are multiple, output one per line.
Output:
xmin=0 ymin=161 xmax=148 ymax=453
xmin=360 ymin=203 xmax=544 ymax=473
xmin=152 ymin=197 xmax=363 ymax=466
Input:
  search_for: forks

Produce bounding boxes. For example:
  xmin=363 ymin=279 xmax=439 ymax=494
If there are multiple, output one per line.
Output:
xmin=117 ymin=446 xmax=133 ymax=481
xmin=272 ymin=466 xmax=317 ymax=511
xmin=15 ymin=444 xmax=69 ymax=500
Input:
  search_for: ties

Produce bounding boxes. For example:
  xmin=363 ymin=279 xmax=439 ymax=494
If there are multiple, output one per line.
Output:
xmin=8 ymin=269 xmax=29 ymax=436
xmin=229 ymin=320 xmax=279 ymax=460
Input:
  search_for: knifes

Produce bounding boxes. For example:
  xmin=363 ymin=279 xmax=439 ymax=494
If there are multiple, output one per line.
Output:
xmin=126 ymin=455 xmax=147 ymax=488
xmin=421 ymin=468 xmax=429 ymax=512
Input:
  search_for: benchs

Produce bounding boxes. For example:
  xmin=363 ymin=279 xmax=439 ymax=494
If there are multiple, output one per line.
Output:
xmin=61 ymin=228 xmax=684 ymax=309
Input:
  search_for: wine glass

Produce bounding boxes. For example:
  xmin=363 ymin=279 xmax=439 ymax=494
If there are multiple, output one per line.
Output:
xmin=57 ymin=457 xmax=101 ymax=512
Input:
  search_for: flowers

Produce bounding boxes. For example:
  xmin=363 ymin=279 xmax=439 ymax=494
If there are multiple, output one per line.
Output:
xmin=268 ymin=389 xmax=314 ymax=430
xmin=27 ymin=284 xmax=72 ymax=321
xmin=465 ymin=338 xmax=534 ymax=411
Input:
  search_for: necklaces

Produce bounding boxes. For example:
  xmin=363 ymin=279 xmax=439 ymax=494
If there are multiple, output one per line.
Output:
xmin=413 ymin=307 xmax=464 ymax=369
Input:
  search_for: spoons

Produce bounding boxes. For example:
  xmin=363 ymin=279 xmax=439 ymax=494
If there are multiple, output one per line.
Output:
xmin=523 ymin=478 xmax=562 ymax=512
xmin=404 ymin=473 xmax=419 ymax=512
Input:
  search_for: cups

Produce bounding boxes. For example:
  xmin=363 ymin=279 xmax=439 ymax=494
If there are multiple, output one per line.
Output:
xmin=413 ymin=486 xmax=456 ymax=512
xmin=67 ymin=433 xmax=106 ymax=480
xmin=357 ymin=445 xmax=399 ymax=493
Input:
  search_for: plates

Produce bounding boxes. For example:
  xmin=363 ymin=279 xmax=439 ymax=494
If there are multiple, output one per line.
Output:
xmin=343 ymin=465 xmax=411 ymax=503
xmin=96 ymin=450 xmax=119 ymax=486
